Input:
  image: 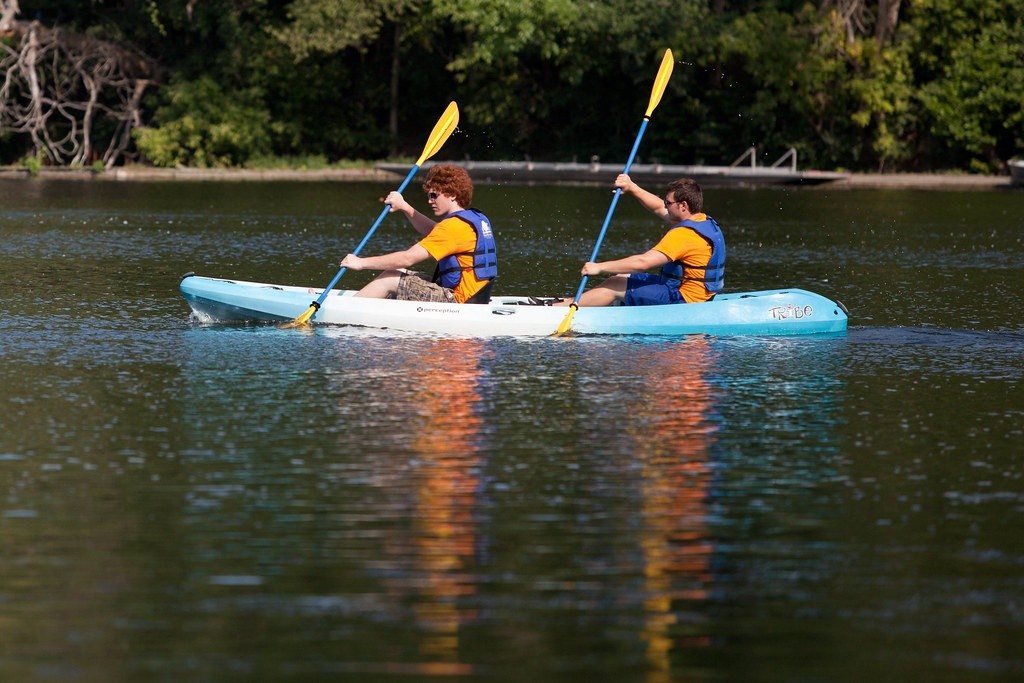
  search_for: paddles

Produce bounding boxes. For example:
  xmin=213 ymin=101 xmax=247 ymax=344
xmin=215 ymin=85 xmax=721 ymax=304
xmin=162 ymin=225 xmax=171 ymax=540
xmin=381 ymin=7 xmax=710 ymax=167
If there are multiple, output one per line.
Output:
xmin=557 ymin=45 xmax=678 ymax=340
xmin=293 ymin=97 xmax=465 ymax=329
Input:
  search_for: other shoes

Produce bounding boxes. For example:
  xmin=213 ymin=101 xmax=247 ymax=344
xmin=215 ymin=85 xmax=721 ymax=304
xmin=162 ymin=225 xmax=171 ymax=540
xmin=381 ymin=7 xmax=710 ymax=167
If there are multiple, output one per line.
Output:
xmin=309 ymin=289 xmax=322 ymax=295
xmin=517 ymin=301 xmax=549 ymax=309
xmin=528 ymin=296 xmax=560 ymax=306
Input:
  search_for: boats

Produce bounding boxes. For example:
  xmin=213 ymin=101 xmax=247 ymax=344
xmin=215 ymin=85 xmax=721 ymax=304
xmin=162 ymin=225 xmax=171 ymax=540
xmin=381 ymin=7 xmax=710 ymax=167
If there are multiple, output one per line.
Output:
xmin=178 ymin=271 xmax=852 ymax=338
xmin=1006 ymin=158 xmax=1024 ymax=184
xmin=376 ymin=148 xmax=849 ymax=189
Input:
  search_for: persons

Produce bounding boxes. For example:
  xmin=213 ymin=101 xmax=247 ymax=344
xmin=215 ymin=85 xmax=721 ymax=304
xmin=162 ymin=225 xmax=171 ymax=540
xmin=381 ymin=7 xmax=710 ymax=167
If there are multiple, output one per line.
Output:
xmin=529 ymin=172 xmax=726 ymax=307
xmin=307 ymin=164 xmax=497 ymax=304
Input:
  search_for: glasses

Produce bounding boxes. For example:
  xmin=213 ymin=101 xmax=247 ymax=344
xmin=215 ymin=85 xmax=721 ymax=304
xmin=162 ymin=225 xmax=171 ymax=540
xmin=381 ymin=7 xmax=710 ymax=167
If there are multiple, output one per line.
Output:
xmin=426 ymin=191 xmax=443 ymax=200
xmin=664 ymin=198 xmax=688 ymax=208
